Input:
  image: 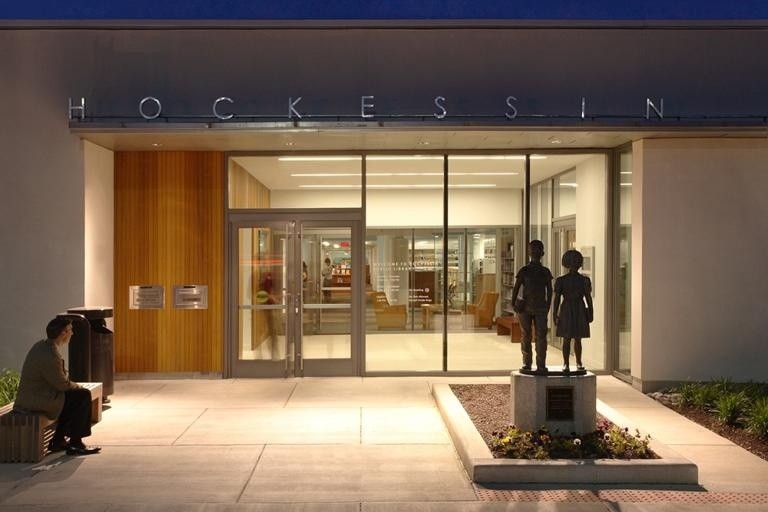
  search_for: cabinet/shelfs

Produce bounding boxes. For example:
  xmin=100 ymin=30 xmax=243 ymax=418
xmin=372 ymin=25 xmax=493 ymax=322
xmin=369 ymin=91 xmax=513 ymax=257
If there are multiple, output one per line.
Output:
xmin=330 ymin=266 xmax=352 ymax=288
xmin=500 ymin=255 xmax=517 ymax=315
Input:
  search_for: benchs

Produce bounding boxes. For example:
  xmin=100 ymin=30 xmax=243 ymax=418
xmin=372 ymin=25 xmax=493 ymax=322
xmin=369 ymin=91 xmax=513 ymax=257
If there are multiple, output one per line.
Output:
xmin=0 ymin=381 xmax=104 ymax=464
xmin=494 ymin=316 xmax=522 ymax=344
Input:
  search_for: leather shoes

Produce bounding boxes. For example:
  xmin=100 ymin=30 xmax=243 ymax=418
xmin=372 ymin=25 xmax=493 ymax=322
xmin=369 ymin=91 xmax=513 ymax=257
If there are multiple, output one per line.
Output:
xmin=66 ymin=444 xmax=102 ymax=455
xmin=48 ymin=440 xmax=70 ymax=451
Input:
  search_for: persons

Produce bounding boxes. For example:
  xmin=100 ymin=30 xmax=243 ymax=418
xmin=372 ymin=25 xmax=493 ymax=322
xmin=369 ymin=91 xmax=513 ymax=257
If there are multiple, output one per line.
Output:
xmin=303 ymin=261 xmax=309 ymax=312
xmin=553 ymin=250 xmax=593 ymax=371
xmin=511 ymin=240 xmax=553 ymax=374
xmin=13 ymin=318 xmax=101 ymax=455
xmin=321 ymin=257 xmax=333 ymax=297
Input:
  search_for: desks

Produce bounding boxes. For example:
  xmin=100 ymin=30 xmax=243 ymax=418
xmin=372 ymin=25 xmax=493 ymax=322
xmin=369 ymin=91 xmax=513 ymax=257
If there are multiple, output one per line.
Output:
xmin=420 ymin=303 xmax=452 ymax=331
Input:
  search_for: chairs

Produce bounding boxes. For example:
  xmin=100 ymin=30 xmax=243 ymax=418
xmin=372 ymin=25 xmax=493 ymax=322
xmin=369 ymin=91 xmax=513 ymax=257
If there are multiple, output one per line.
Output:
xmin=367 ymin=289 xmax=408 ymax=332
xmin=459 ymin=290 xmax=501 ymax=331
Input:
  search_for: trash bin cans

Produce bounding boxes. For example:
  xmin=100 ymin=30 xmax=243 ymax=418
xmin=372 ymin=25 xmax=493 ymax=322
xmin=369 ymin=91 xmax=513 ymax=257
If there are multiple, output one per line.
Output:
xmin=66 ymin=306 xmax=114 ymax=405
